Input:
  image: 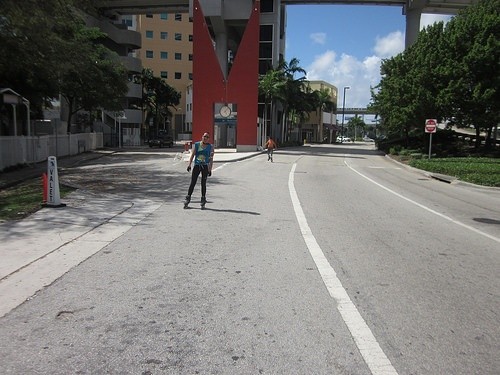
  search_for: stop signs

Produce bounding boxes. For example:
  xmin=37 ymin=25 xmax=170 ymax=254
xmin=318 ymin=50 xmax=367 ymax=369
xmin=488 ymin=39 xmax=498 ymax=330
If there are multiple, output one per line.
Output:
xmin=425 ymin=119 xmax=437 ymax=133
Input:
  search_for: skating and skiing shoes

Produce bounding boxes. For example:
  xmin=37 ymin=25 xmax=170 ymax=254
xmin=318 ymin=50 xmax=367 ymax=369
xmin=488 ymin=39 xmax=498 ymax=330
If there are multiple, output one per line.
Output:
xmin=183 ymin=195 xmax=191 ymax=209
xmin=200 ymin=196 xmax=207 ymax=209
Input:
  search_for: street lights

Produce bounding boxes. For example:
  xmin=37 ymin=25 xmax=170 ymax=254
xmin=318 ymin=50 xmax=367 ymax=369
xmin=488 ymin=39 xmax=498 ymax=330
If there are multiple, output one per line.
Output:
xmin=341 ymin=87 xmax=350 ymax=143
xmin=118 ymin=111 xmax=127 ymax=149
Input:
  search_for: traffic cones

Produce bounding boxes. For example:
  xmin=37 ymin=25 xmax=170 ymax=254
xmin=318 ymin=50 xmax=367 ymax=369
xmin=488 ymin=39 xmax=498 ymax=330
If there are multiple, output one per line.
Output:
xmin=39 ymin=155 xmax=66 ymax=208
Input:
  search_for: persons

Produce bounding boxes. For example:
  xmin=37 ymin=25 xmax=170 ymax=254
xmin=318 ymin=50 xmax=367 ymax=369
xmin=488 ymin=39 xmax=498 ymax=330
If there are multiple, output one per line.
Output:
xmin=265 ymin=137 xmax=277 ymax=161
xmin=183 ymin=132 xmax=215 ymax=206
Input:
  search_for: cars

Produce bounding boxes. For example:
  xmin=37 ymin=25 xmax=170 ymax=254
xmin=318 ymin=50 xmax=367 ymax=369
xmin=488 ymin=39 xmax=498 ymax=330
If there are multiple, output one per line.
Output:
xmin=337 ymin=135 xmax=350 ymax=142
xmin=148 ymin=135 xmax=173 ymax=148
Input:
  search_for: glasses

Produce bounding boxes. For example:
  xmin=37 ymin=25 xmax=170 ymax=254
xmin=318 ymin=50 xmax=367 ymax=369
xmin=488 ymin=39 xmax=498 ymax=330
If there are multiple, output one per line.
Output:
xmin=202 ymin=135 xmax=210 ymax=138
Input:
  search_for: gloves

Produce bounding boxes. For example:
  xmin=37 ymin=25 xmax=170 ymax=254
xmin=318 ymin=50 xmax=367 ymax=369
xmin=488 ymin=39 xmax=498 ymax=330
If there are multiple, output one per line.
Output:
xmin=208 ymin=170 xmax=211 ymax=176
xmin=187 ymin=166 xmax=191 ymax=172
